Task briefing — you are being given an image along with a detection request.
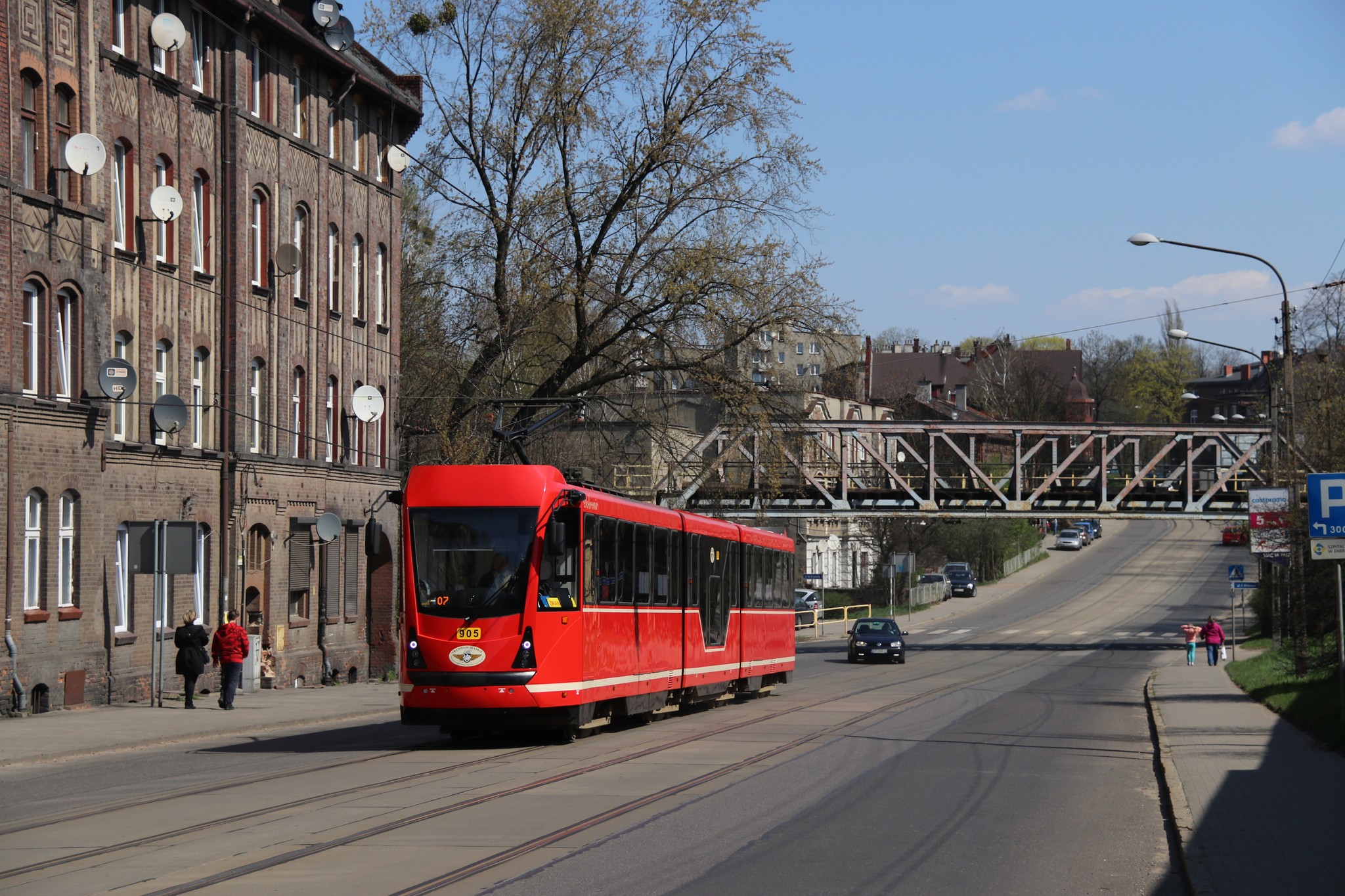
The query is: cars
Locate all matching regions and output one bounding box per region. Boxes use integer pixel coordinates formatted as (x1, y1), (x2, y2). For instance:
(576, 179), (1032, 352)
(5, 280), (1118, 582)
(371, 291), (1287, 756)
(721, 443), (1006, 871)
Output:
(1055, 529), (1082, 550)
(1069, 519), (1102, 546)
(795, 595), (815, 631)
(946, 570), (977, 597)
(917, 572), (952, 601)
(1221, 525), (1249, 546)
(847, 617), (909, 664)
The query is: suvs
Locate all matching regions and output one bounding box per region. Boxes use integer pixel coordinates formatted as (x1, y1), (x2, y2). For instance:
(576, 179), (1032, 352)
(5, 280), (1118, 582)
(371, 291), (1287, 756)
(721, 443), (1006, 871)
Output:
(795, 588), (823, 619)
(942, 561), (974, 576)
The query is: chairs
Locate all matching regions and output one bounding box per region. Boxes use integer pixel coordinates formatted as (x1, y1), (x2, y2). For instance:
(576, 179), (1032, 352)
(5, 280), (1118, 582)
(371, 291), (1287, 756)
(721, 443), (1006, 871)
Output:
(860, 624), (869, 631)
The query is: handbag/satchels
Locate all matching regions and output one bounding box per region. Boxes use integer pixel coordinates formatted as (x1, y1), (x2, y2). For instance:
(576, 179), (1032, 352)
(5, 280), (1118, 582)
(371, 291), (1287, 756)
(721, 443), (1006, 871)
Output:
(201, 647), (210, 665)
(1220, 642), (1227, 660)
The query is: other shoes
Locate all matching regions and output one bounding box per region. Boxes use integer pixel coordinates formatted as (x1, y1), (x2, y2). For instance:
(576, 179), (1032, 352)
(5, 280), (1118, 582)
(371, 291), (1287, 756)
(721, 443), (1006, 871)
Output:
(1209, 663), (1213, 666)
(1214, 663), (1217, 665)
(1188, 662), (1191, 665)
(218, 697), (226, 708)
(1191, 662), (1194, 666)
(185, 704), (196, 708)
(225, 704), (235, 710)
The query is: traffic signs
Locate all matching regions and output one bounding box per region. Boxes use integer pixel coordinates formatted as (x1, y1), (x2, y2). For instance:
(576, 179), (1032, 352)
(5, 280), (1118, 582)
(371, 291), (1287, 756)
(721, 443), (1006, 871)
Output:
(1307, 473), (1345, 538)
(1228, 564), (1244, 580)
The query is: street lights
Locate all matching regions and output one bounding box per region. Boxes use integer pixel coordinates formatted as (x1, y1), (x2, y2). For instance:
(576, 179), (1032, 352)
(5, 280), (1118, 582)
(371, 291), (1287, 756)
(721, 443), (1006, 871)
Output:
(1128, 232), (1310, 682)
(1169, 329), (1280, 651)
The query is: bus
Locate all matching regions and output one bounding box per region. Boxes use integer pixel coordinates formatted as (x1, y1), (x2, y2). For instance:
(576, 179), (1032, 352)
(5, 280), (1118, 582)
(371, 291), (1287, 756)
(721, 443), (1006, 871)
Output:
(365, 396), (796, 746)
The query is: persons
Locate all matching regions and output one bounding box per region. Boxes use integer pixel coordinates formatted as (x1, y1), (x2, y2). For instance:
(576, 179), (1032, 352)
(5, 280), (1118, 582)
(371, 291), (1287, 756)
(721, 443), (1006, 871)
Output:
(476, 552), (511, 588)
(211, 609), (249, 710)
(1181, 624), (1202, 666)
(1200, 615), (1225, 666)
(174, 609), (210, 709)
(876, 623), (894, 635)
